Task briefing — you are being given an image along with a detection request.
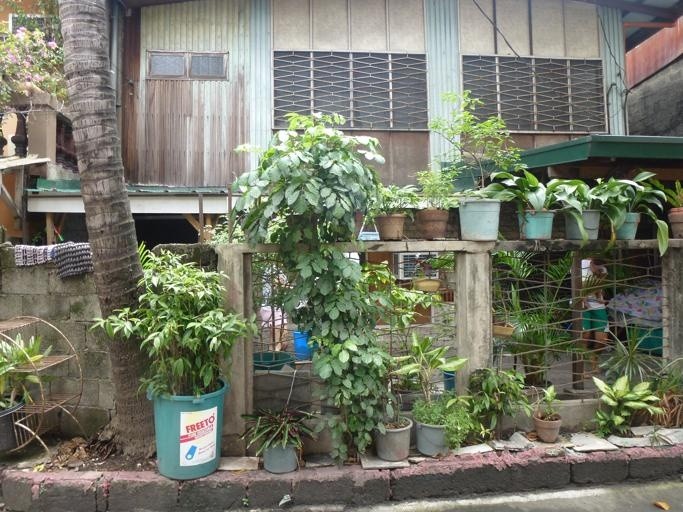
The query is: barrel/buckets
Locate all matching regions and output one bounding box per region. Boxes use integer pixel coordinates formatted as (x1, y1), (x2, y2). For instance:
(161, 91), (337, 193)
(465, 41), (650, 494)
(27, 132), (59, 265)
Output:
(443, 370), (455, 392)
(373, 416), (413, 462)
(564, 209), (601, 240)
(0, 394), (27, 459)
(413, 416), (450, 458)
(458, 198), (501, 242)
(152, 377), (228, 481)
(263, 442), (299, 474)
(293, 331), (321, 362)
(252, 350), (296, 373)
(515, 209), (558, 240)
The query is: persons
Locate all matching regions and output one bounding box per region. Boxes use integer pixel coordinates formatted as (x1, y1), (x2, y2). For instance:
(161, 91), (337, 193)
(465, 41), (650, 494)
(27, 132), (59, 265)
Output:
(573, 258), (607, 376)
(579, 259), (610, 310)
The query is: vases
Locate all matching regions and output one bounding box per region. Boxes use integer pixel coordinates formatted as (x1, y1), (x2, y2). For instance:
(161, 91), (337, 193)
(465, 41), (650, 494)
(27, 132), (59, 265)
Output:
(442, 369), (455, 391)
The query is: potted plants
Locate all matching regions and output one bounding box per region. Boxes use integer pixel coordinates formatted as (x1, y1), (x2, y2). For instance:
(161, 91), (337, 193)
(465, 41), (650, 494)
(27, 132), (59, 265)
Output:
(586, 171), (670, 258)
(490, 161), (590, 240)
(84, 237), (261, 483)
(427, 89), (526, 242)
(409, 163), (464, 239)
(1, 329), (66, 455)
(531, 384), (562, 444)
(412, 390), (476, 458)
(664, 179), (681, 239)
(551, 175), (603, 240)
(238, 402), (325, 477)
(368, 182), (419, 240)
(348, 163), (379, 240)
(365, 347), (422, 461)
